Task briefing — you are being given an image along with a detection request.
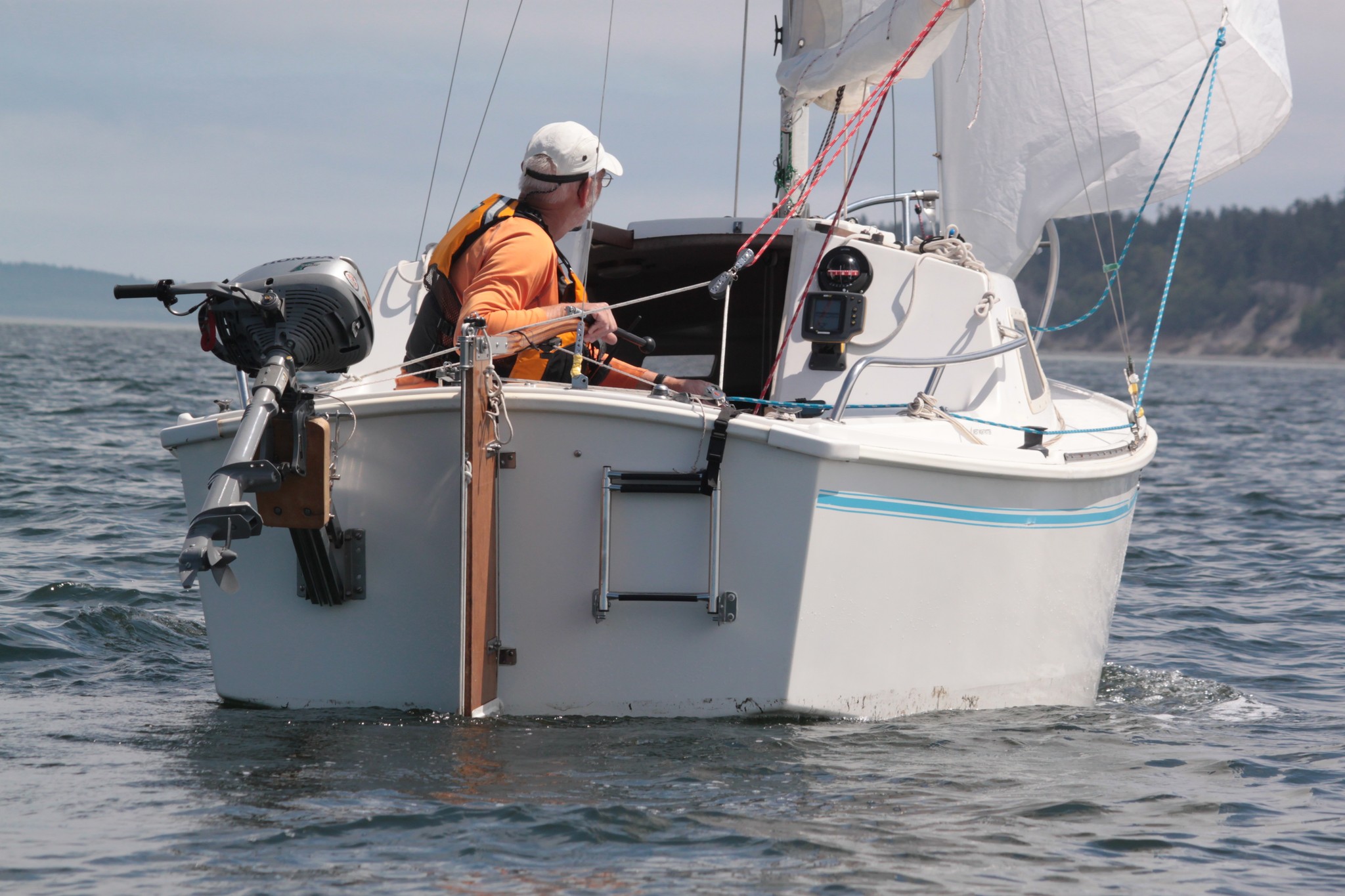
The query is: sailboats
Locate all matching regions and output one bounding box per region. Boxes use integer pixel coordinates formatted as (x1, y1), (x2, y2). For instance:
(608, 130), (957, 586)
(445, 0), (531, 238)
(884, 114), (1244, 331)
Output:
(114, 0), (1294, 722)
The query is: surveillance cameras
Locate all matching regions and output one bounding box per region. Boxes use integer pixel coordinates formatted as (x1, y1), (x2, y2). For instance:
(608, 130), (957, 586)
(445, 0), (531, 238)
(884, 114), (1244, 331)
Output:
(818, 246), (873, 295)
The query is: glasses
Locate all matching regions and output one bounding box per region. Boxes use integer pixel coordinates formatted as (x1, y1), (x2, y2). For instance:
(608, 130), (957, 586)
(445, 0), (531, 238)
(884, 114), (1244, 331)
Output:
(577, 171), (613, 199)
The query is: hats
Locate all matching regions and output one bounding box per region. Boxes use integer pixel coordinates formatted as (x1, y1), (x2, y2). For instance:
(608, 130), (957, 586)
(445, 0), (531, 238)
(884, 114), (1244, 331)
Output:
(520, 121), (624, 184)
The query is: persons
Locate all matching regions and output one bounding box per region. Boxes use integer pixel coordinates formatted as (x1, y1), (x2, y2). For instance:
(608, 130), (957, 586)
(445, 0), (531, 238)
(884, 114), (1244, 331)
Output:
(394, 121), (734, 413)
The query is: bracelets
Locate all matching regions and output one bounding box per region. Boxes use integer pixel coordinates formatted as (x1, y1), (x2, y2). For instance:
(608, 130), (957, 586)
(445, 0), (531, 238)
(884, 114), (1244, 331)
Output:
(653, 373), (668, 388)
